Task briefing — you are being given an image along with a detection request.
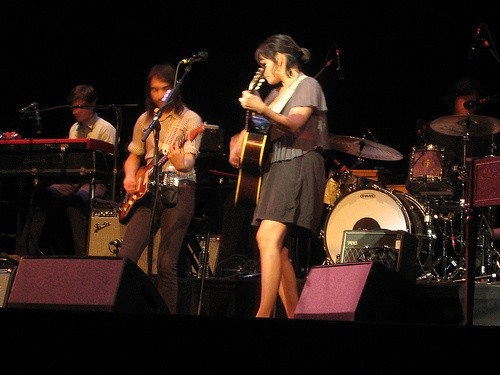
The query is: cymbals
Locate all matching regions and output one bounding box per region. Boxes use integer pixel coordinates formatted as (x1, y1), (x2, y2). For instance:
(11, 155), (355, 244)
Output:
(327, 134), (405, 162)
(430, 113), (500, 136)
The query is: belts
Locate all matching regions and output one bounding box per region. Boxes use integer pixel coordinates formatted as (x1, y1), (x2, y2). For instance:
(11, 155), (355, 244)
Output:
(179, 180), (195, 189)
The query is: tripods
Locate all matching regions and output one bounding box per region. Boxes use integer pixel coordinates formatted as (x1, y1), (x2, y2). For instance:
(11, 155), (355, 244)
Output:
(416, 186), (466, 282)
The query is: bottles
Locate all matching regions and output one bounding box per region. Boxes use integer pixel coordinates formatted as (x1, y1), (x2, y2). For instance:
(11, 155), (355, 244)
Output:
(163, 166), (179, 186)
(197, 246), (209, 278)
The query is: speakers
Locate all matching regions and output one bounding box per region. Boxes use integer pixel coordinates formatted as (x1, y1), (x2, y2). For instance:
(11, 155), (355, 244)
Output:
(191, 185), (252, 271)
(87, 208), (161, 276)
(0, 269), (12, 307)
(339, 230), (419, 283)
(7, 255), (170, 315)
(293, 261), (413, 323)
(185, 234), (235, 277)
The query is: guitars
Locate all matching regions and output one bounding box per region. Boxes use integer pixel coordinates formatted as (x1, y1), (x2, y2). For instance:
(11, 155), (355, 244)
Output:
(233, 62), (274, 208)
(118, 121), (208, 224)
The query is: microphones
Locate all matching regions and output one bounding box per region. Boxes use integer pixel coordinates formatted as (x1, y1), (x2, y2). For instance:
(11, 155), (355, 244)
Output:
(416, 122), (425, 143)
(464, 96), (491, 110)
(180, 52), (208, 64)
(336, 48), (344, 79)
(19, 102), (38, 113)
(472, 26), (481, 50)
(109, 239), (123, 247)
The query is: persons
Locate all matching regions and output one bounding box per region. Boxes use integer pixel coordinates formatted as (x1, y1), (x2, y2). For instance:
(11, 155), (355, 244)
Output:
(427, 82), (486, 160)
(118, 65), (203, 315)
(230, 34), (327, 318)
(8, 84), (120, 256)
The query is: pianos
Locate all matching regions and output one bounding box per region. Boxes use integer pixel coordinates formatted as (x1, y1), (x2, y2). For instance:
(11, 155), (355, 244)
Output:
(0, 137), (116, 257)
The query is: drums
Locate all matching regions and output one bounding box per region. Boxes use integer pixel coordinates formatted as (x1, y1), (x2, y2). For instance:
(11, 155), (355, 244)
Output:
(407, 143), (463, 198)
(322, 168), (373, 207)
(322, 185), (434, 278)
